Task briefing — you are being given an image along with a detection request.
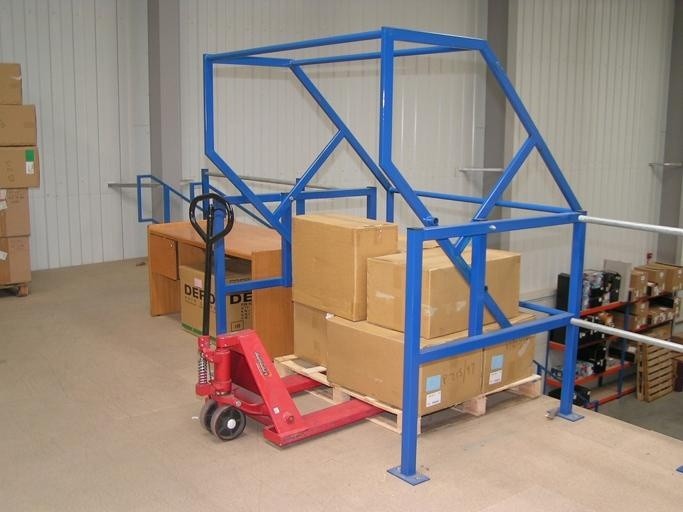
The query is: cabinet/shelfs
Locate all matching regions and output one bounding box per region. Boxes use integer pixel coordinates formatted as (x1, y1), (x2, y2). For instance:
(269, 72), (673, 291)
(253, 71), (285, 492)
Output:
(546, 292), (677, 410)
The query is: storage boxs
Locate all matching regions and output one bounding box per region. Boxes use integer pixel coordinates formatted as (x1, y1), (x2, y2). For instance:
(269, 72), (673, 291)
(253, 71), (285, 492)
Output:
(178, 259), (252, 344)
(292, 213), (536, 416)
(0, 63), (40, 291)
(607, 262), (683, 350)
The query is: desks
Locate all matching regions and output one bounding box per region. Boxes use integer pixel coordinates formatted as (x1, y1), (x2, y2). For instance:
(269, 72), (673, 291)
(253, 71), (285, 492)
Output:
(147, 220), (294, 362)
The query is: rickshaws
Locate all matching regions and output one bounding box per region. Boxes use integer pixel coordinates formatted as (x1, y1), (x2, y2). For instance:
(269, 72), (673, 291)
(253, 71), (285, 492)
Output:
(188, 193), (387, 448)
